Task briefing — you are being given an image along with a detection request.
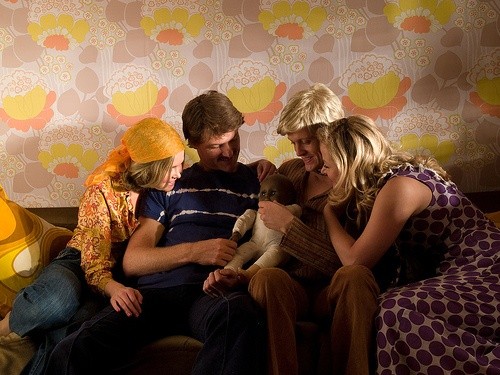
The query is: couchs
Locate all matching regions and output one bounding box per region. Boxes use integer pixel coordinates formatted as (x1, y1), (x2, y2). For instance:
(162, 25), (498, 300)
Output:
(25, 190), (500, 375)
